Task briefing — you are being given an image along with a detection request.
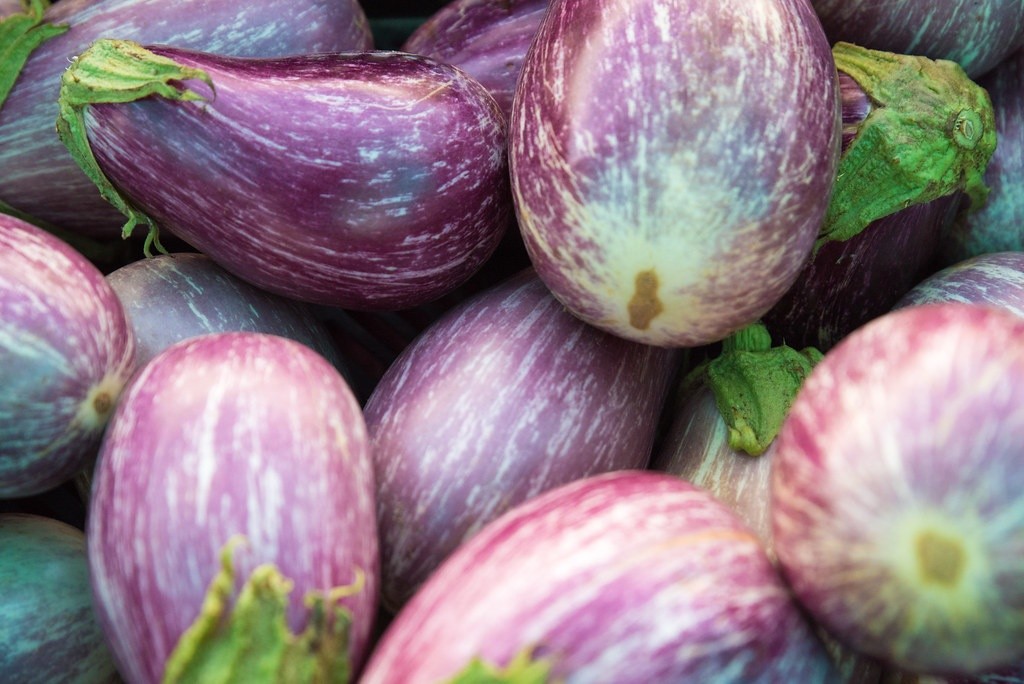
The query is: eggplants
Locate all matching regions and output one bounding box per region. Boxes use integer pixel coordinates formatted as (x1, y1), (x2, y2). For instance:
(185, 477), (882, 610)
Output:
(0, 0), (1024, 684)
